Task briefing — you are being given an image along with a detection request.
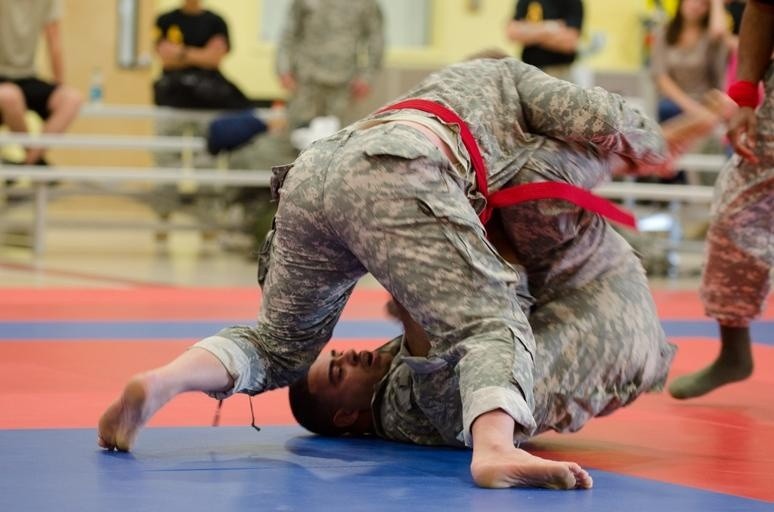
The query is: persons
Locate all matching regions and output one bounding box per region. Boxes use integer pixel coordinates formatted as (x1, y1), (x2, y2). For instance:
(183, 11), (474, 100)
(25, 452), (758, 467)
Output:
(142, 0), (234, 266)
(506, 0), (584, 82)
(708, 0), (766, 162)
(269, 0), (385, 132)
(0, 1), (84, 191)
(96, 44), (673, 493)
(284, 94), (734, 449)
(666, 0), (773, 400)
(641, 0), (731, 125)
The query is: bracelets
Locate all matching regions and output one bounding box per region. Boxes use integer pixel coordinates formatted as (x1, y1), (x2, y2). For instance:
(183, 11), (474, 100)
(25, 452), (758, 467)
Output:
(724, 79), (760, 109)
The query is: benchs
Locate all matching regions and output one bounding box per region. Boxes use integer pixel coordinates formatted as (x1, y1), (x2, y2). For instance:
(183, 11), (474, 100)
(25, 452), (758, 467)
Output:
(593, 154), (725, 245)
(1, 105), (285, 252)
